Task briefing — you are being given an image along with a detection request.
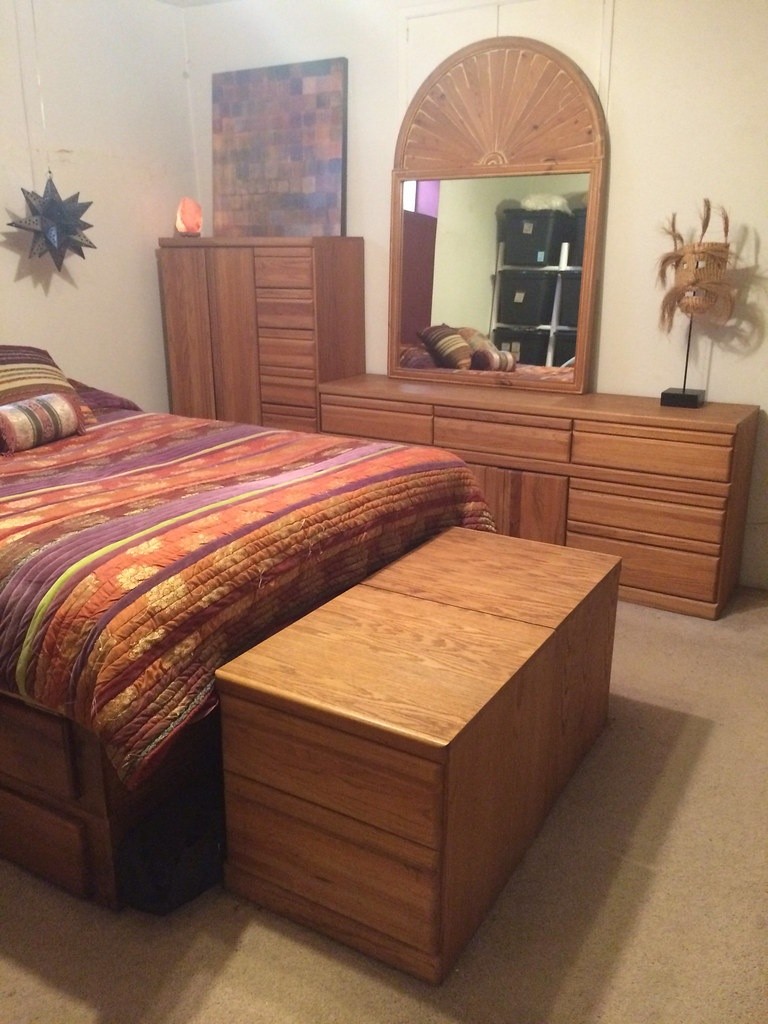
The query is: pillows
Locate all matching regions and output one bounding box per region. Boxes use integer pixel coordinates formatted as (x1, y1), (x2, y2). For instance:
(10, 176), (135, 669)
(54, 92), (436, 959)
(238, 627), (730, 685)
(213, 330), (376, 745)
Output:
(0, 394), (88, 455)
(400, 343), (439, 370)
(0, 344), (97, 427)
(471, 349), (516, 372)
(418, 323), (473, 370)
(454, 327), (498, 353)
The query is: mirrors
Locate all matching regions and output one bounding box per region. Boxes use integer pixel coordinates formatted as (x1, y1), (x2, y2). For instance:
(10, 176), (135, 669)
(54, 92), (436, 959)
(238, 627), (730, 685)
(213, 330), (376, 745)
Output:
(385, 162), (606, 396)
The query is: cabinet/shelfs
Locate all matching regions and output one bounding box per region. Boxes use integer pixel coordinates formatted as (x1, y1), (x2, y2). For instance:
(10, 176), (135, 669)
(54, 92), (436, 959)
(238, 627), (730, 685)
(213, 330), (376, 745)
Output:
(320, 371), (760, 618)
(155, 234), (364, 435)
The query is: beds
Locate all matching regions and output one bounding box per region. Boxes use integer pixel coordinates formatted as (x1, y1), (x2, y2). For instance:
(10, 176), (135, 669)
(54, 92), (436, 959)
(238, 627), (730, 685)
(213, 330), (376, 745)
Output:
(0, 375), (498, 917)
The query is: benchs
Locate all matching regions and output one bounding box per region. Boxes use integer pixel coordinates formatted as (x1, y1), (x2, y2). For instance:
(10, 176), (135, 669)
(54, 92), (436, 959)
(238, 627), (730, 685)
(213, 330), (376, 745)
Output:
(215, 525), (621, 987)
